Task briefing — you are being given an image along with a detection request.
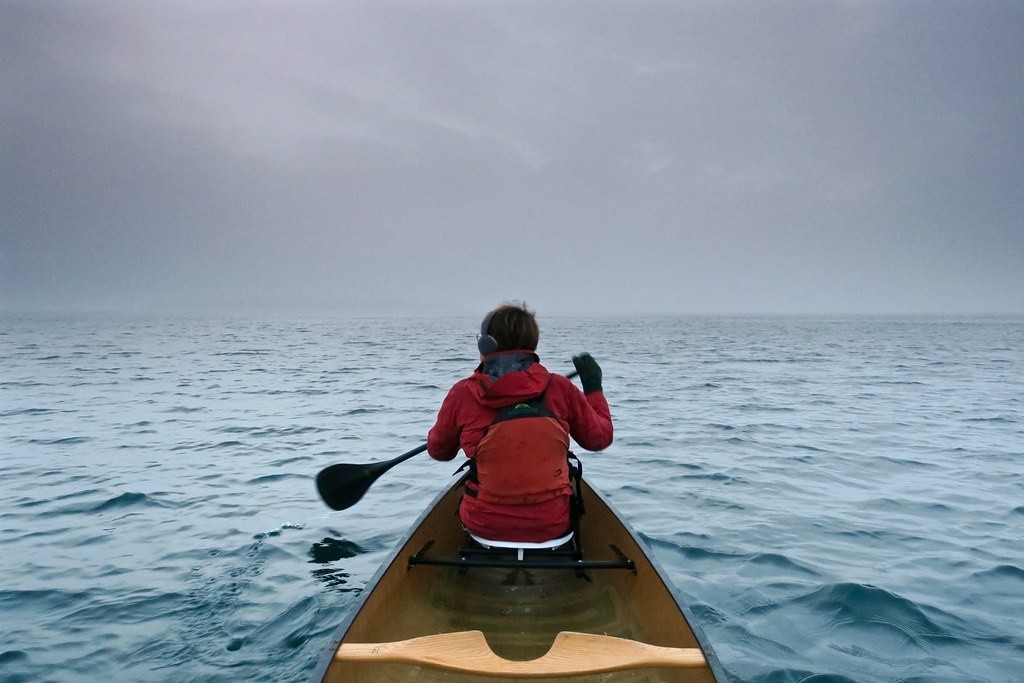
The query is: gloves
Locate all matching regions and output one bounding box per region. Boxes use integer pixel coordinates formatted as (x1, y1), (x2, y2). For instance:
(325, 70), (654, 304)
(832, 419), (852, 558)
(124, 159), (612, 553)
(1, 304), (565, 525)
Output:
(573, 351), (602, 393)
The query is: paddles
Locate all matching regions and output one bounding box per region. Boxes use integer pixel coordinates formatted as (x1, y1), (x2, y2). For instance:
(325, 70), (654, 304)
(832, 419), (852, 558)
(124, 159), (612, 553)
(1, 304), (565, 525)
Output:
(310, 367), (582, 515)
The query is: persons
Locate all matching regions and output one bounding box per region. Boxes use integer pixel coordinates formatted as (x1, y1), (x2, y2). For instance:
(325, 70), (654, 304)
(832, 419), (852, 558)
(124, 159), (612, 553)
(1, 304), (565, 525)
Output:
(427, 305), (613, 554)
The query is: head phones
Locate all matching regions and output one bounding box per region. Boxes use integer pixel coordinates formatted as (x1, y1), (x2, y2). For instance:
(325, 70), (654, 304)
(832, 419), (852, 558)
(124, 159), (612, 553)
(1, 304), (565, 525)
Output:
(478, 309), (497, 356)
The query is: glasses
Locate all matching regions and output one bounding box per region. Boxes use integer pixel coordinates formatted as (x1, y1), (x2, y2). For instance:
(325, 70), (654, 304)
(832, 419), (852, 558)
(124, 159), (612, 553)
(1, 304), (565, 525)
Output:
(476, 333), (483, 342)
